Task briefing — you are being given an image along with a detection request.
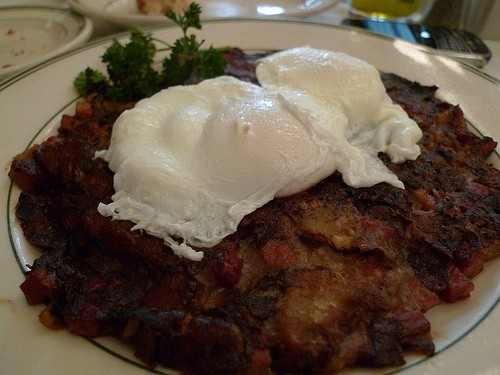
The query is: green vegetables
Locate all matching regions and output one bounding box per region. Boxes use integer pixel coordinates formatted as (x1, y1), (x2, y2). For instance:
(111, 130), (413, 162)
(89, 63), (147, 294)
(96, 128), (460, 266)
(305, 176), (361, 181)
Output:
(75, 2), (237, 98)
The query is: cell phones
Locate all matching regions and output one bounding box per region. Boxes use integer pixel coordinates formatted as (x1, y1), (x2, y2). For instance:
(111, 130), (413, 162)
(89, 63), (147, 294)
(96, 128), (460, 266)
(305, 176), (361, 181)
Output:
(342, 18), (492, 68)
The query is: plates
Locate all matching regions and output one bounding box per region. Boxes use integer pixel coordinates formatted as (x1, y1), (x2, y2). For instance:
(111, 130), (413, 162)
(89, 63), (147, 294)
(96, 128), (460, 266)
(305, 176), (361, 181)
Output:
(1, 0), (92, 78)
(72, 0), (351, 29)
(0, 18), (499, 374)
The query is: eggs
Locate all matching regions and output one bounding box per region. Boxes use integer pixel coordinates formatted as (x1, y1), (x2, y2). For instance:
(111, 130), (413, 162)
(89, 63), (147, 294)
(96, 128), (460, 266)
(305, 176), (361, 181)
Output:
(255, 49), (421, 163)
(94, 76), (404, 261)
